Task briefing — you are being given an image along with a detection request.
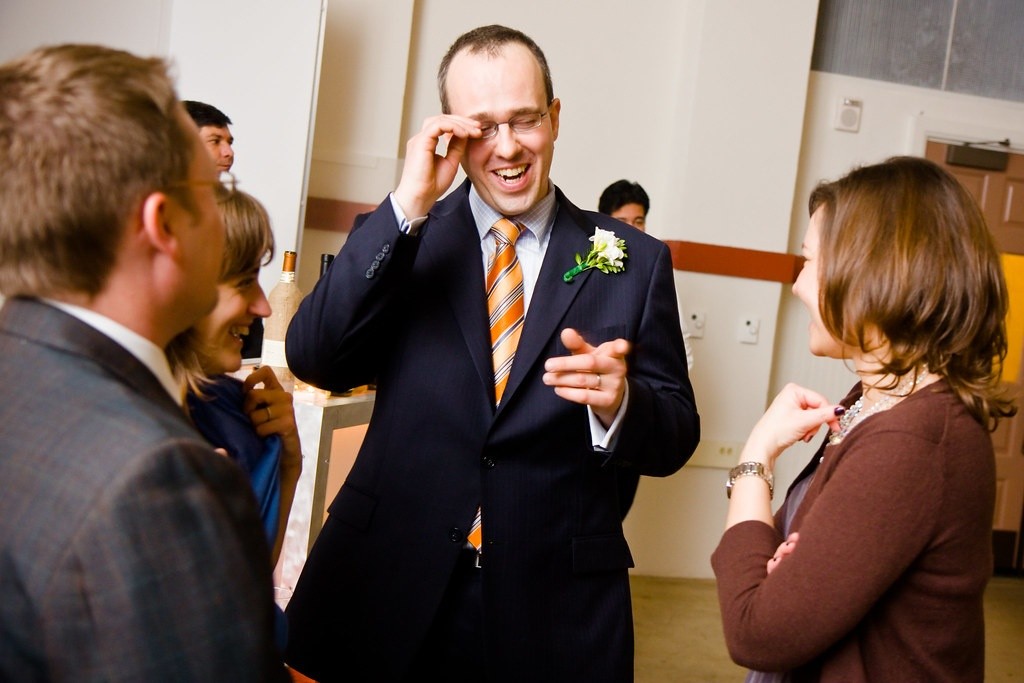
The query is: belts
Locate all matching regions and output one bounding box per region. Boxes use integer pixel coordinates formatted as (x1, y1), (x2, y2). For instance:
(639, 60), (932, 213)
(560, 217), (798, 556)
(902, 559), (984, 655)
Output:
(452, 547), (492, 571)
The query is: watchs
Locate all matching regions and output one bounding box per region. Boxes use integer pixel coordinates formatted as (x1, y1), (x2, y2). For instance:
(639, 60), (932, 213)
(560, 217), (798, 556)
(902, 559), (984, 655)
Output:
(726, 462), (774, 502)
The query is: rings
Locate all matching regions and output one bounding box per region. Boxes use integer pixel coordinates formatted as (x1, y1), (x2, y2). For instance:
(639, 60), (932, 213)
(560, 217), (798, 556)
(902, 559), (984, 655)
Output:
(594, 373), (601, 391)
(266, 407), (272, 422)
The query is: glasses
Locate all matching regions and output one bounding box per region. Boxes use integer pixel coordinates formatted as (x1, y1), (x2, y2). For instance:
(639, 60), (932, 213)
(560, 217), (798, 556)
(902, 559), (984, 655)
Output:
(136, 167), (239, 233)
(479, 101), (554, 138)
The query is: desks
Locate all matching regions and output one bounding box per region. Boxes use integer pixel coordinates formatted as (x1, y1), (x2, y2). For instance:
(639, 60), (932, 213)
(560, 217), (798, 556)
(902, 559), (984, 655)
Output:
(234, 368), (375, 613)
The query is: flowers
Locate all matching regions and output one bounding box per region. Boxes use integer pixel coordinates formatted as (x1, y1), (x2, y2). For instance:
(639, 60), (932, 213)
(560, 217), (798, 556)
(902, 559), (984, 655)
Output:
(563, 225), (629, 282)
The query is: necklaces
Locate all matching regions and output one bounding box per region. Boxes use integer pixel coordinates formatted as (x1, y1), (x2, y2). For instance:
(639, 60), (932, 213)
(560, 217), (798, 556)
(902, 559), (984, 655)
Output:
(818, 363), (930, 445)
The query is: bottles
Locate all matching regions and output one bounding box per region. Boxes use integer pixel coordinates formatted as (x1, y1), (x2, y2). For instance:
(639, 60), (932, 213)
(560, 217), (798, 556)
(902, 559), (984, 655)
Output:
(260, 250), (306, 394)
(320, 253), (334, 278)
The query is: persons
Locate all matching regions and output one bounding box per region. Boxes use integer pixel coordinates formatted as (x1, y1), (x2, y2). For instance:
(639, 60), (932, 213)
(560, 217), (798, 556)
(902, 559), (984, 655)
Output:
(0, 45), (287, 683)
(597, 179), (650, 234)
(180, 100), (264, 383)
(710, 156), (1017, 683)
(283, 25), (701, 683)
(163, 184), (303, 683)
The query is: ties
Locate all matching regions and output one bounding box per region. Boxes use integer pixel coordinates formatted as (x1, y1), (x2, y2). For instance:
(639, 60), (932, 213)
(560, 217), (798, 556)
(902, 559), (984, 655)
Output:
(466, 218), (527, 555)
